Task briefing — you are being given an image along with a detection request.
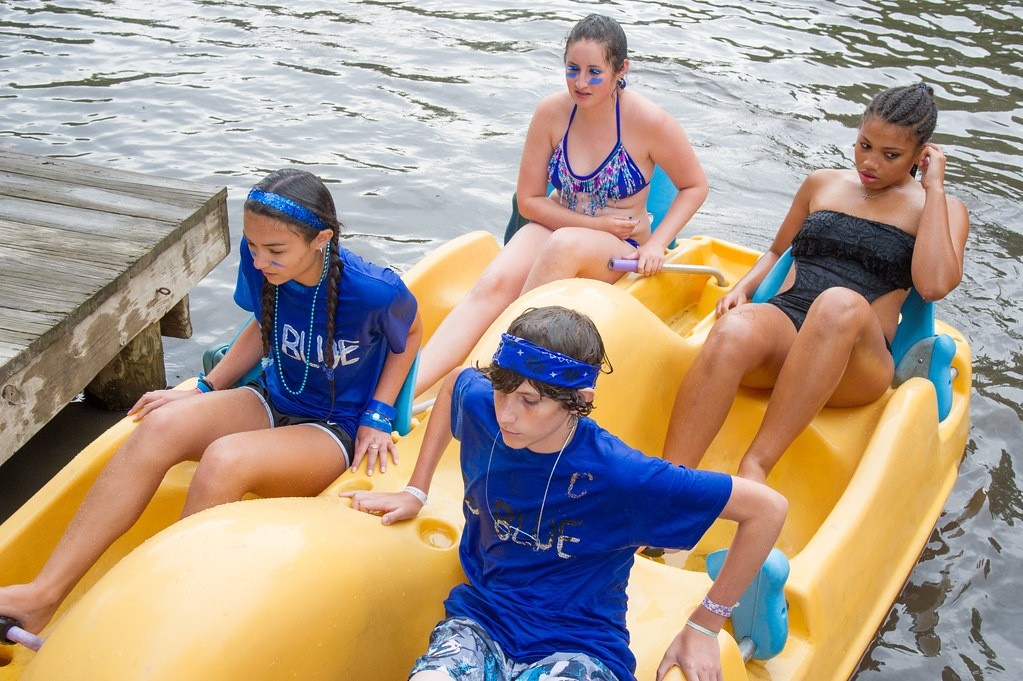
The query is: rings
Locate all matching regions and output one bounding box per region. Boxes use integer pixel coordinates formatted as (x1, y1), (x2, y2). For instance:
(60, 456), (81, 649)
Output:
(369, 445), (379, 449)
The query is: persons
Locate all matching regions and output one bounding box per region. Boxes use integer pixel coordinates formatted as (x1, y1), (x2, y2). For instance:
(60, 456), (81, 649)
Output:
(636, 79), (968, 555)
(412, 13), (709, 402)
(340, 306), (788, 681)
(0, 169), (423, 636)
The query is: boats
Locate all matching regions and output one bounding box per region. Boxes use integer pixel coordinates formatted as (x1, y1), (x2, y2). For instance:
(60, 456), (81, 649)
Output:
(1, 230), (972, 680)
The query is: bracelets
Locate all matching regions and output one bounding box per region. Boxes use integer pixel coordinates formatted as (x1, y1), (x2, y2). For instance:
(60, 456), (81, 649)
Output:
(685, 620), (719, 638)
(196, 378), (215, 394)
(359, 400), (398, 433)
(404, 486), (427, 504)
(702, 597), (733, 617)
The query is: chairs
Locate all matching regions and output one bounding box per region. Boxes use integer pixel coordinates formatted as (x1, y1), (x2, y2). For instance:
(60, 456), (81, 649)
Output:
(535, 161), (681, 257)
(225, 310), (417, 434)
(754, 246), (956, 425)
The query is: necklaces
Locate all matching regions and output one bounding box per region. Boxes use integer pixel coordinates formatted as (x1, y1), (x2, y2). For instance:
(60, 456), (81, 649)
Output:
(486, 418), (577, 550)
(275, 241), (330, 395)
(861, 184), (901, 202)
